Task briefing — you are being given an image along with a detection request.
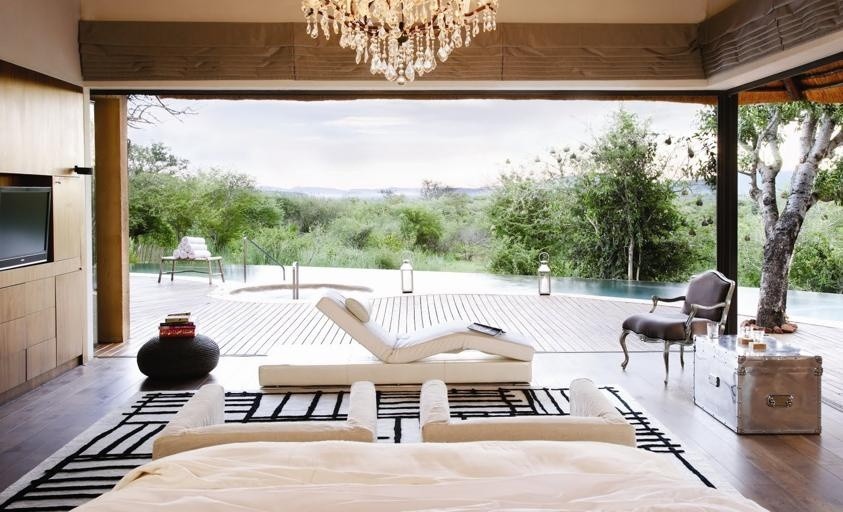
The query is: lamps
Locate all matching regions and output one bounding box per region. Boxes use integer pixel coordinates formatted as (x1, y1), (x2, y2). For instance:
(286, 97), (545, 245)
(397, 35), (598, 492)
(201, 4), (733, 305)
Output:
(298, 0), (500, 90)
(71, 165), (95, 176)
(535, 251), (552, 296)
(399, 260), (414, 294)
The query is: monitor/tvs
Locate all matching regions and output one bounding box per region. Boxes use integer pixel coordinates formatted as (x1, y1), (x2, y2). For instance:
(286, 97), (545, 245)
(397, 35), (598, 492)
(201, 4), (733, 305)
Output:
(0, 186), (52, 271)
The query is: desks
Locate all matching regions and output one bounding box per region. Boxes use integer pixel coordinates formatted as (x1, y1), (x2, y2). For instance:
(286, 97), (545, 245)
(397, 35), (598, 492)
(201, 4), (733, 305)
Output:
(155, 256), (224, 288)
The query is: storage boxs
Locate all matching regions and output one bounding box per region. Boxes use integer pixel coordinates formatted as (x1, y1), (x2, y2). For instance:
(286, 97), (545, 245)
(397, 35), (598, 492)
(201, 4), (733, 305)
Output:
(684, 331), (827, 438)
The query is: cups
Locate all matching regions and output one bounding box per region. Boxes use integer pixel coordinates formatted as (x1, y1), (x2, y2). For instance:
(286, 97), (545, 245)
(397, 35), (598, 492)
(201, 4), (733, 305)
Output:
(706, 322), (765, 346)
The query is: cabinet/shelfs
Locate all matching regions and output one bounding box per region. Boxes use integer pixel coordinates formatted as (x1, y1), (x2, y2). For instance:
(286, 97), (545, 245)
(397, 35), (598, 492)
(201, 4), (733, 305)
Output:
(0, 258), (85, 396)
(22, 176), (82, 262)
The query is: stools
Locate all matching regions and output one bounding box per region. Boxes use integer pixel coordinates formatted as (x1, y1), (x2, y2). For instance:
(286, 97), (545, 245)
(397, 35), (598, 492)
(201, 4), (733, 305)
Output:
(134, 334), (219, 381)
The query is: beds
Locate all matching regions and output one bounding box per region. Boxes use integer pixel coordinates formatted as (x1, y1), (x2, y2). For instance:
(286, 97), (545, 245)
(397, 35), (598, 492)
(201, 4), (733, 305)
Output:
(65, 434), (767, 512)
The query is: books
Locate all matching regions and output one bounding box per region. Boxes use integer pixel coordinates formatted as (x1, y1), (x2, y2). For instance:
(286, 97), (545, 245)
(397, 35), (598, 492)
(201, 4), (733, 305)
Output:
(466, 322), (508, 338)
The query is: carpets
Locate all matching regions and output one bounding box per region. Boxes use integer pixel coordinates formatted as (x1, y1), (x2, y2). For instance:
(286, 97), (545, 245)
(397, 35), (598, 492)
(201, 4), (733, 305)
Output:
(0, 383), (744, 512)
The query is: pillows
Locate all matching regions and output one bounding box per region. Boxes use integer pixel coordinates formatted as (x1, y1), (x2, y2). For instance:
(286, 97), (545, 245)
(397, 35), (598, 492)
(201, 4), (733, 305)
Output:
(343, 292), (371, 324)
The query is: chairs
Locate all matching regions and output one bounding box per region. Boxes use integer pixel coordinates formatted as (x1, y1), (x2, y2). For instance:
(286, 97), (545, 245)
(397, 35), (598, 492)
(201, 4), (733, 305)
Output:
(149, 379), (381, 460)
(614, 267), (735, 391)
(413, 373), (638, 449)
(257, 290), (536, 387)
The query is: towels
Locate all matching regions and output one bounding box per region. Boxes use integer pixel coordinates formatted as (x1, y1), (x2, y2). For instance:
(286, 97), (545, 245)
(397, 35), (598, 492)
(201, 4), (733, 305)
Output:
(171, 235), (211, 261)
